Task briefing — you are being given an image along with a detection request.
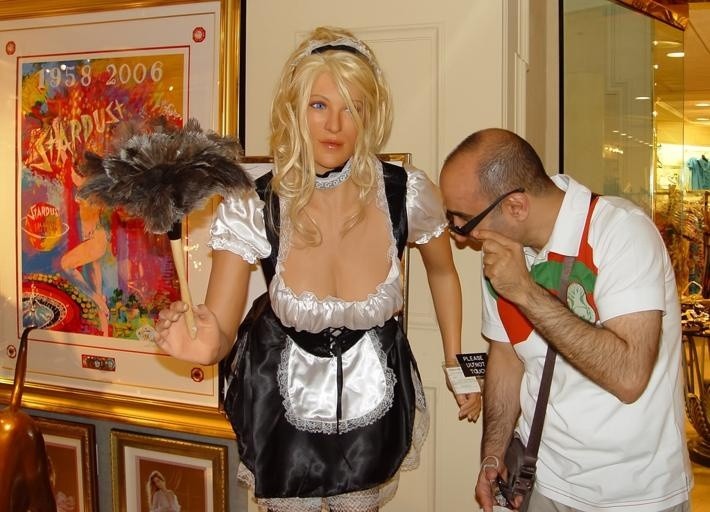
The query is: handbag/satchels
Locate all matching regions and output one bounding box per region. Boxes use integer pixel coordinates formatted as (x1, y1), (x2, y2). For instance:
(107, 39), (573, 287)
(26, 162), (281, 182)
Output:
(490, 436), (548, 512)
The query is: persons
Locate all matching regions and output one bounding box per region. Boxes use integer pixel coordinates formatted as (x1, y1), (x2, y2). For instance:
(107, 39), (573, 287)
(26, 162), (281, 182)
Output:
(439, 128), (695, 512)
(147, 470), (181, 512)
(52, 118), (110, 335)
(153, 26), (481, 512)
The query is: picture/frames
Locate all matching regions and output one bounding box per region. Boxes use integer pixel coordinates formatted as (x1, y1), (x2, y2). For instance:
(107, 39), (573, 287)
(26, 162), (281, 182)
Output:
(106, 430), (231, 512)
(1, 0), (245, 442)
(28, 414), (98, 512)
(241, 154), (414, 338)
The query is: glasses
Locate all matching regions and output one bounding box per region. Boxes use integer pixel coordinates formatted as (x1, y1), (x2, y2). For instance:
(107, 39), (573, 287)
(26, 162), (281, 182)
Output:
(445, 186), (527, 238)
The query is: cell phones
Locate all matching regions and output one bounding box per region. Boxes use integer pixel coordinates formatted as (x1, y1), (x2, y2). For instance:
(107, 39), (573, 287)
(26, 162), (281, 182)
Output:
(492, 473), (516, 509)
